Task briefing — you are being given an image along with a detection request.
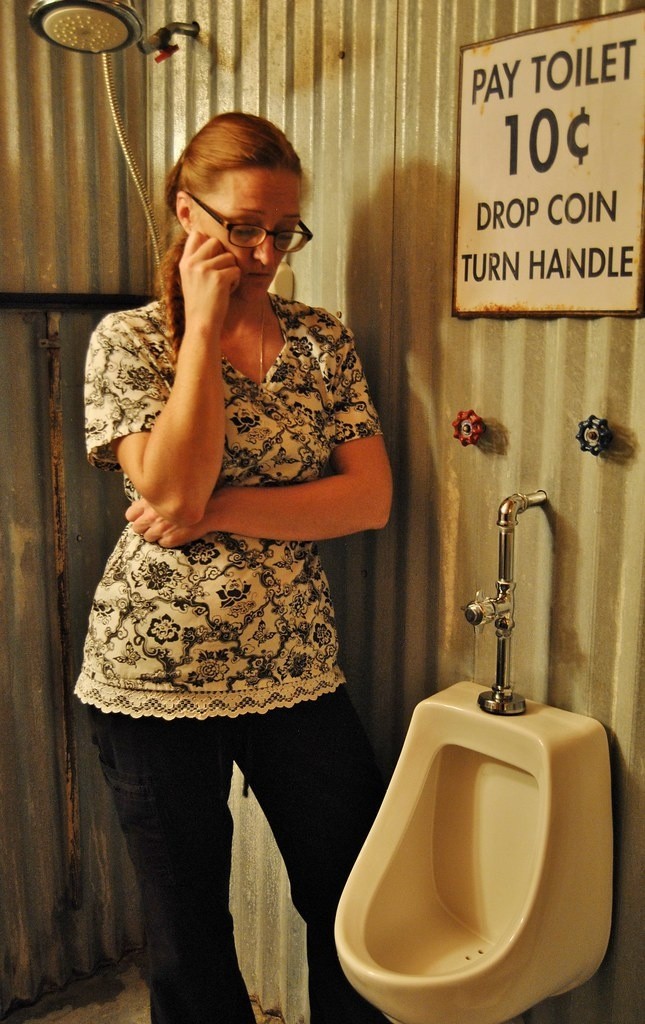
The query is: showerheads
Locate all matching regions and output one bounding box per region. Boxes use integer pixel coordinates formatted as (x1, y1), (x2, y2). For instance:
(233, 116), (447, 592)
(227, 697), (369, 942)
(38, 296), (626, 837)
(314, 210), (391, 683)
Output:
(29, 0), (145, 55)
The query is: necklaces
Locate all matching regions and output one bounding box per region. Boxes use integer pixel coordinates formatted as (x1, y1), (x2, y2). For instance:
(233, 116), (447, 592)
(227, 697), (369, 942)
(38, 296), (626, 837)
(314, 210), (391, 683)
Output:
(220, 293), (265, 414)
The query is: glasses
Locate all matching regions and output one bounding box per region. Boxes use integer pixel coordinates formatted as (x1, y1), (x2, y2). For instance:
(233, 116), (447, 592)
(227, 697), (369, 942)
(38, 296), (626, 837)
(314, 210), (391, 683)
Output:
(186, 191), (313, 254)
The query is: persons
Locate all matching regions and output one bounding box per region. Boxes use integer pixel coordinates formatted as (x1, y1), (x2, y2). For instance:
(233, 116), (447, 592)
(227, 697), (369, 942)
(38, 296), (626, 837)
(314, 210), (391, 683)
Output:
(74, 113), (392, 1024)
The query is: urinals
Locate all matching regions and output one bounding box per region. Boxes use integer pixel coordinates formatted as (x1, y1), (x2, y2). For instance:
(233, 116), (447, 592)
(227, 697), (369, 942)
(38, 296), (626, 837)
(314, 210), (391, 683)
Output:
(335, 682), (614, 1017)
